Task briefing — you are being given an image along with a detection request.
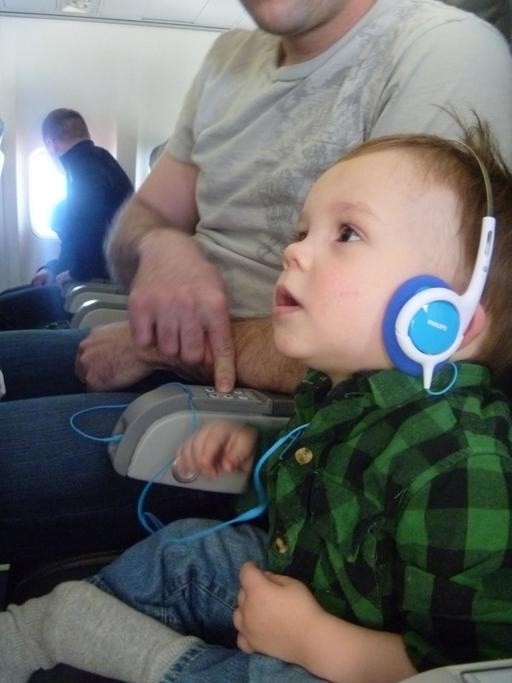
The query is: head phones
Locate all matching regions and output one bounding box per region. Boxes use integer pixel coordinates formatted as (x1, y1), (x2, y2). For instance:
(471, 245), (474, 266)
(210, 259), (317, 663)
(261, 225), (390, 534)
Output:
(382, 140), (497, 390)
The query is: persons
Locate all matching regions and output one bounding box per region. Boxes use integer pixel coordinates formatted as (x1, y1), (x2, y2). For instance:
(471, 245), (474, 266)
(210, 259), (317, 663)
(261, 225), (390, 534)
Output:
(0, 97), (512, 683)
(1, 106), (135, 326)
(0, 2), (512, 552)
